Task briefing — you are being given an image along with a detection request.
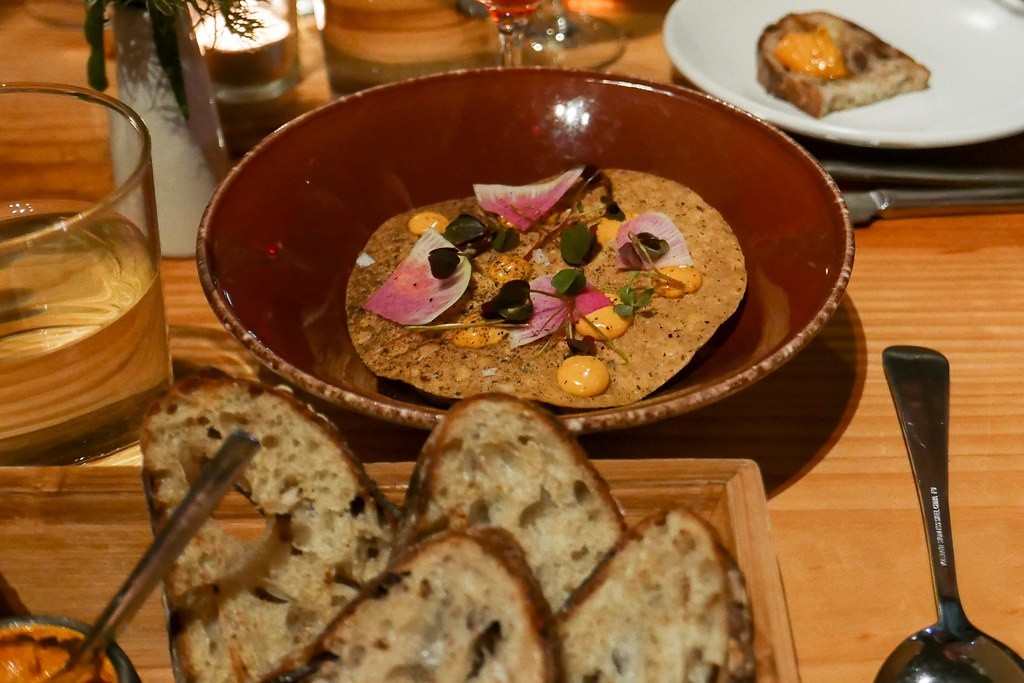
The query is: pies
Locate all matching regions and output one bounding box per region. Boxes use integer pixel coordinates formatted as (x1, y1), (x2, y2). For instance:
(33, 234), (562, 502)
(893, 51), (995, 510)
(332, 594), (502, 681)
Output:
(343, 167), (747, 411)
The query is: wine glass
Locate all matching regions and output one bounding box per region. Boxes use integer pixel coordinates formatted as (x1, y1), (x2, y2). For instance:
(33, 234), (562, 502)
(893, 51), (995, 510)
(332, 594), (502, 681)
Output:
(502, 0), (626, 77)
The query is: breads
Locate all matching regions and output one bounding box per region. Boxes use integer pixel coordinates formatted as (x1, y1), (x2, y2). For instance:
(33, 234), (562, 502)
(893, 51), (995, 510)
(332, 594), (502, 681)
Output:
(139, 376), (756, 683)
(755, 10), (931, 118)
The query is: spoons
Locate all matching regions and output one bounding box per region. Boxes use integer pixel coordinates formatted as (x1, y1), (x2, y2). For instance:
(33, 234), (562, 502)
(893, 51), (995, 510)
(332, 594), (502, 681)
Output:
(873, 344), (1024, 683)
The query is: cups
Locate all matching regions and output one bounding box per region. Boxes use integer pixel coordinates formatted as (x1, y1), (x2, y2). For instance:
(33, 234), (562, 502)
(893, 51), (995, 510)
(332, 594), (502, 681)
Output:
(1, 81), (176, 466)
(173, 0), (304, 98)
(321, 0), (506, 102)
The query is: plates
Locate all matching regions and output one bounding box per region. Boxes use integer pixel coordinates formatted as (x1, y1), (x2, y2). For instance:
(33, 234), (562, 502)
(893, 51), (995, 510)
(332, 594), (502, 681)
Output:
(661, 0), (1024, 149)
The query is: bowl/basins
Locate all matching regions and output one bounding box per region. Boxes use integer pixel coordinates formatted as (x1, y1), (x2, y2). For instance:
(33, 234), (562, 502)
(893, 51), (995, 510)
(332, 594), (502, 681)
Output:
(195, 66), (855, 432)
